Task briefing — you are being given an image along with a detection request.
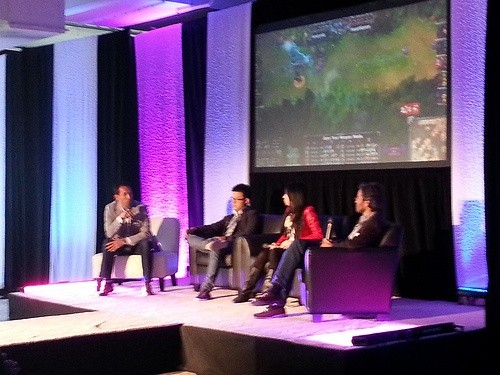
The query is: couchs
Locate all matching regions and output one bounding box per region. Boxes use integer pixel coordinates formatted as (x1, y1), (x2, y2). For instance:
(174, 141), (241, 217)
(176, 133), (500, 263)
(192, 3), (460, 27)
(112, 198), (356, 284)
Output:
(186, 212), (405, 322)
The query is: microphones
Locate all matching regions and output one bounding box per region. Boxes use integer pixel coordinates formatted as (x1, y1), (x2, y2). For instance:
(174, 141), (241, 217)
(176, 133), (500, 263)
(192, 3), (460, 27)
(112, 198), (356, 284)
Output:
(325, 219), (332, 239)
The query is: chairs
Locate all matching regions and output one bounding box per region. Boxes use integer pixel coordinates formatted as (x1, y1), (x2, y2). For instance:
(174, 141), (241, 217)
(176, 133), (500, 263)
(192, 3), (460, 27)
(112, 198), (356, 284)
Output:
(92, 217), (180, 292)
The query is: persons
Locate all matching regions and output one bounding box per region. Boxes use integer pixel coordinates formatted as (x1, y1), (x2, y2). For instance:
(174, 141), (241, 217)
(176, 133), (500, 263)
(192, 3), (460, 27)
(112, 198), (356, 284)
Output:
(233, 185), (323, 302)
(186, 183), (260, 301)
(250, 183), (386, 319)
(97, 185), (157, 296)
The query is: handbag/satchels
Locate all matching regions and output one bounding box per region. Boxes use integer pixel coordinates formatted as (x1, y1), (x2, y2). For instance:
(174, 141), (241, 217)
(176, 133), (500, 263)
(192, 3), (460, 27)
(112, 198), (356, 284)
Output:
(115, 221), (163, 256)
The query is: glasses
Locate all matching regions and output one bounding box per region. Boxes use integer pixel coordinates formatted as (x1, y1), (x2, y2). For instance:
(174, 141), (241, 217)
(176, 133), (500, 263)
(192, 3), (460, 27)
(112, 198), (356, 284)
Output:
(230, 197), (244, 201)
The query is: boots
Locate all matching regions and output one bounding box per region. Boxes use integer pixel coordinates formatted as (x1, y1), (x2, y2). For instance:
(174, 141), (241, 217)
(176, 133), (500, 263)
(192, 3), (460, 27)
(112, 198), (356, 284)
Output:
(250, 268), (276, 301)
(233, 267), (259, 303)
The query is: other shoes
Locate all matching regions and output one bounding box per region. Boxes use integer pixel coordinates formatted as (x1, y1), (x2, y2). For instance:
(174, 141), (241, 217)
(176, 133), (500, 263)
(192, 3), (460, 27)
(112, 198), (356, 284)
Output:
(197, 290), (211, 301)
(208, 240), (228, 251)
(252, 290), (285, 307)
(98, 280), (113, 296)
(142, 285), (152, 296)
(253, 307), (285, 320)
